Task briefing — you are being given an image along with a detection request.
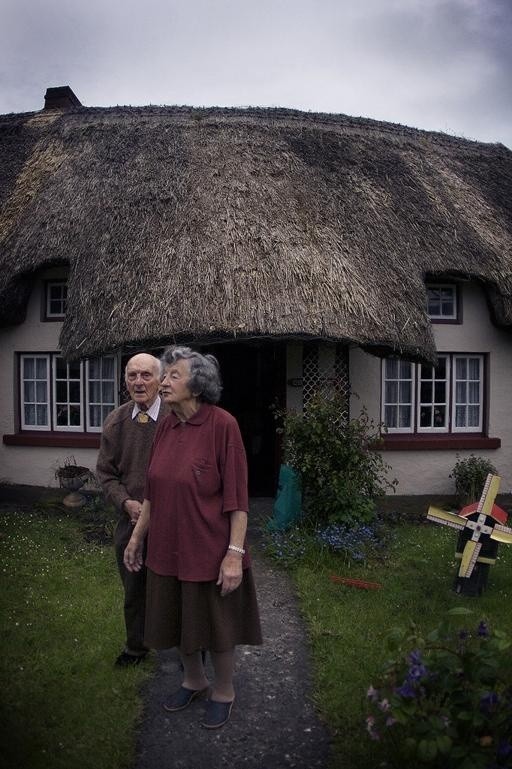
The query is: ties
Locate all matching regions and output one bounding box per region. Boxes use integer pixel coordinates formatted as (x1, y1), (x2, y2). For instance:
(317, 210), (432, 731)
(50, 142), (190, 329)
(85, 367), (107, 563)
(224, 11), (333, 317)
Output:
(136, 412), (150, 424)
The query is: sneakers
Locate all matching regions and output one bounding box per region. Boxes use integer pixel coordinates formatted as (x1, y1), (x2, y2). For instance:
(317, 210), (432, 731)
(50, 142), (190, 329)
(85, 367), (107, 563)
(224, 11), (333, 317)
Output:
(202, 694), (234, 728)
(163, 685), (200, 712)
(115, 644), (148, 668)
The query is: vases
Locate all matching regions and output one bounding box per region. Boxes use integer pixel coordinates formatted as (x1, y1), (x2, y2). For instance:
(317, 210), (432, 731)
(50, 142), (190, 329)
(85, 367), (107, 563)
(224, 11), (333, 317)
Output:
(56, 465), (92, 508)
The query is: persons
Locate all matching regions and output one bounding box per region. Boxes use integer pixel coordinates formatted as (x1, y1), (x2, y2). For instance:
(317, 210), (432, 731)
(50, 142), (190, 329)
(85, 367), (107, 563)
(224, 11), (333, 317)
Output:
(123, 346), (263, 728)
(95, 354), (208, 675)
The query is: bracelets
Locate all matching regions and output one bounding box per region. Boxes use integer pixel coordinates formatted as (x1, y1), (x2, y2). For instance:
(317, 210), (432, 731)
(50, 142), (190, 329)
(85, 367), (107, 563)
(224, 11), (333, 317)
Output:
(228, 544), (246, 555)
